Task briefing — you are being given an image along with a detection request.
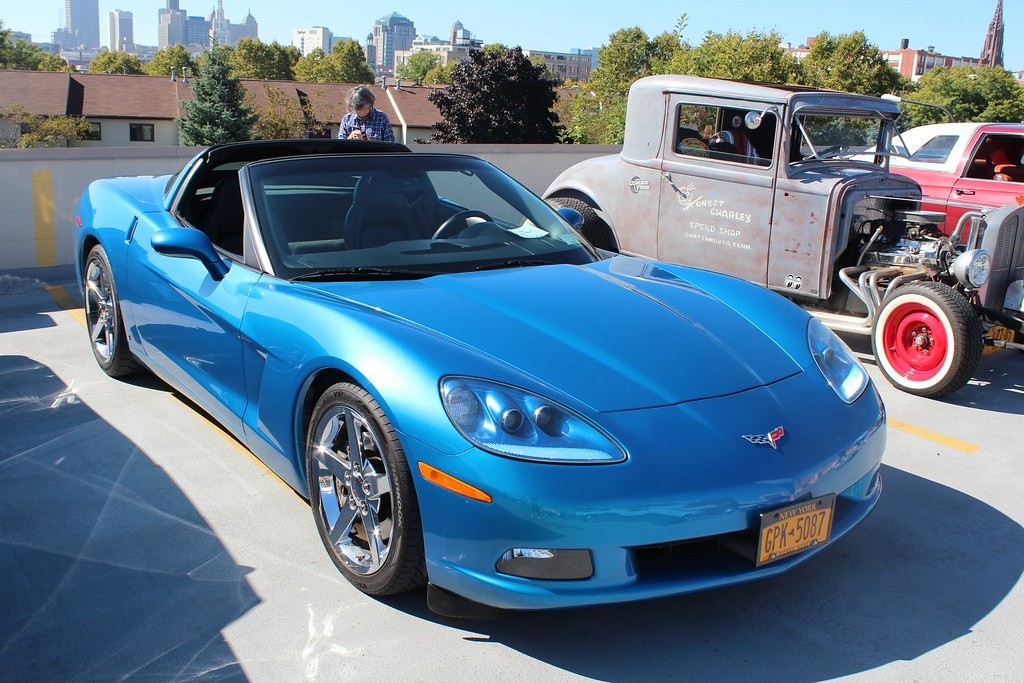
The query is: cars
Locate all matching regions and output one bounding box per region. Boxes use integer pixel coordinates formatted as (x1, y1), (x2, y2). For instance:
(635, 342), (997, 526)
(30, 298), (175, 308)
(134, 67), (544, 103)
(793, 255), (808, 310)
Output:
(519, 73), (1024, 400)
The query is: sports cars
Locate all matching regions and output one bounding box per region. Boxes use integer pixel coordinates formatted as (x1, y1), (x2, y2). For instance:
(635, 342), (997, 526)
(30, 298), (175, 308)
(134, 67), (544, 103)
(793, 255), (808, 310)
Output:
(72, 137), (888, 622)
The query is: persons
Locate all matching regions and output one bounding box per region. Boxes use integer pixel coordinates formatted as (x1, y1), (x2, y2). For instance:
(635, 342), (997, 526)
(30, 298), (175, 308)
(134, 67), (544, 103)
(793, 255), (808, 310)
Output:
(338, 86), (396, 143)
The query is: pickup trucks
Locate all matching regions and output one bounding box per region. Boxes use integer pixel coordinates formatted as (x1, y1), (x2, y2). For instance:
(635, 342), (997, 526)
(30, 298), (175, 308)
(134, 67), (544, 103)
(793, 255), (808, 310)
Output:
(851, 122), (1024, 259)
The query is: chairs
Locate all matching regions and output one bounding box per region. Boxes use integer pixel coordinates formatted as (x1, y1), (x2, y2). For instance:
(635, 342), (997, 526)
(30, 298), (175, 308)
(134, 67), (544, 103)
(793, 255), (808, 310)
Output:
(209, 178), (293, 252)
(994, 164), (1024, 182)
(346, 173), (422, 250)
(680, 127), (760, 165)
